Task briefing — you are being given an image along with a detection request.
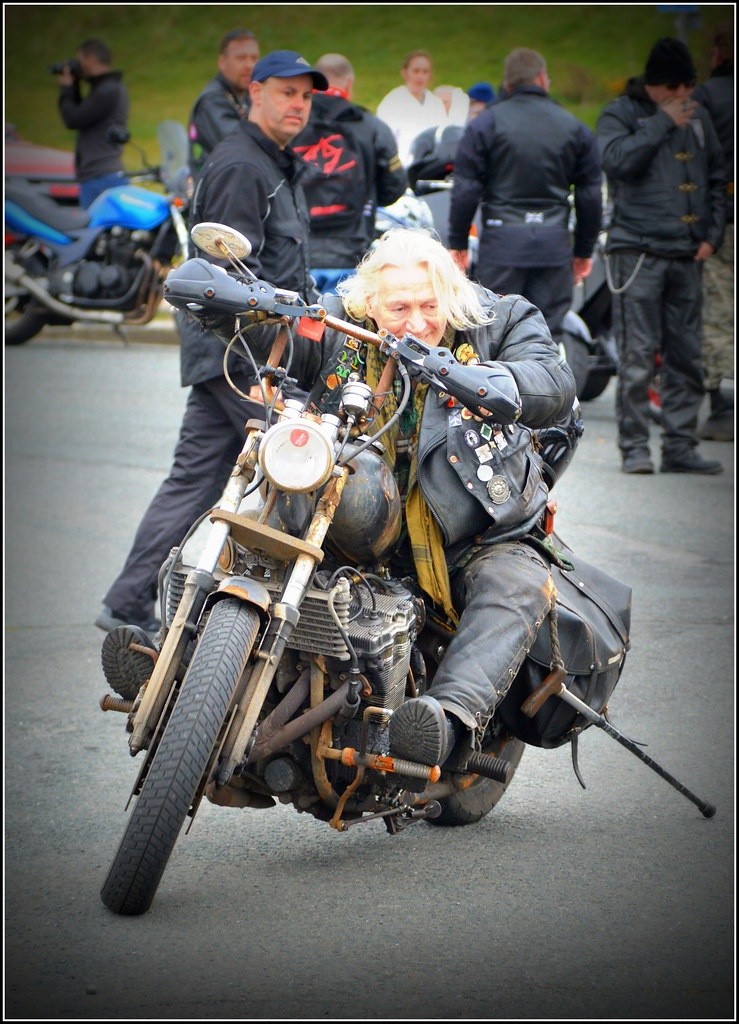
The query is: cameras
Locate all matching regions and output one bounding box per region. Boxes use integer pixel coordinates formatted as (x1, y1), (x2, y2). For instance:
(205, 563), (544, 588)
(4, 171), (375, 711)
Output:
(51, 59), (83, 79)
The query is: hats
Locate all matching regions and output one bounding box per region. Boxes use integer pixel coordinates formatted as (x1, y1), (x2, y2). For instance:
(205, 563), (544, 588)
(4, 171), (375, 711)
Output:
(468, 82), (494, 101)
(642, 37), (699, 85)
(251, 51), (328, 90)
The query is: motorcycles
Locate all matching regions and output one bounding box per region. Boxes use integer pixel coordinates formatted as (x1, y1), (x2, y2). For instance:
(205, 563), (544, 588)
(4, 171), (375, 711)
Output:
(3, 116), (193, 350)
(411, 170), (619, 403)
(98, 220), (587, 916)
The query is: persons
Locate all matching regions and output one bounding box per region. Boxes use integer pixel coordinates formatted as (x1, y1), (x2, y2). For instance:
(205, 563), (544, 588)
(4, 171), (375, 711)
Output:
(88, 24), (311, 645)
(376, 53), (490, 174)
(280, 52), (407, 300)
(448, 43), (605, 330)
(596, 33), (738, 477)
(47, 40), (137, 208)
(101, 228), (582, 795)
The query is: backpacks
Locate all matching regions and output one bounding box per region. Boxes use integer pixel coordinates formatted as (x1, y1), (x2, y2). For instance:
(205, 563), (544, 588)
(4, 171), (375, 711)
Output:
(494, 546), (630, 787)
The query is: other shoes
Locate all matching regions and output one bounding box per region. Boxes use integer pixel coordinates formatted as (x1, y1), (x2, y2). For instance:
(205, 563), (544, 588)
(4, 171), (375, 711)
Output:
(662, 453), (723, 475)
(390, 696), (455, 794)
(102, 624), (159, 702)
(699, 412), (733, 441)
(95, 606), (162, 639)
(621, 447), (656, 474)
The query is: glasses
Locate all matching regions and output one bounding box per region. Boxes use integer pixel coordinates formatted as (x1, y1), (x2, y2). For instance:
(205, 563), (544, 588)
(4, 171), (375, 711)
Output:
(661, 79), (696, 91)
